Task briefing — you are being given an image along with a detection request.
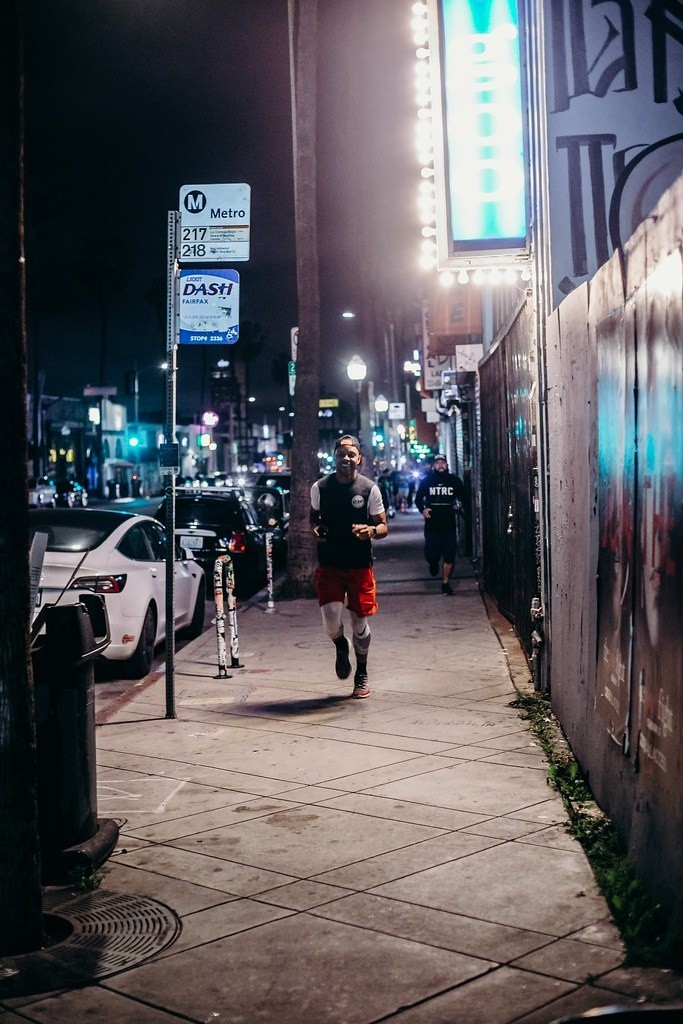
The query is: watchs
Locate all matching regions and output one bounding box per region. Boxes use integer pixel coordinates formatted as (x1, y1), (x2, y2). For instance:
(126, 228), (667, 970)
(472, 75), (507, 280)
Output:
(372, 526), (377, 538)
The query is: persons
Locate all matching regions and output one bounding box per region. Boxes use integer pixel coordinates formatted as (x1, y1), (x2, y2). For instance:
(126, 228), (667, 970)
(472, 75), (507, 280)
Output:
(378, 462), (434, 515)
(414, 454), (467, 595)
(309, 435), (388, 699)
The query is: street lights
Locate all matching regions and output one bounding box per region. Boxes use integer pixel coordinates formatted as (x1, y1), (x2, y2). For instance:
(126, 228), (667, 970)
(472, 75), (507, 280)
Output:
(347, 353), (368, 475)
(374, 394), (389, 470)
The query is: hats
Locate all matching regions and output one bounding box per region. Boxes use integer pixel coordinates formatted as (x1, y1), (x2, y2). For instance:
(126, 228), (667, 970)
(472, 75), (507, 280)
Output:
(434, 454), (447, 463)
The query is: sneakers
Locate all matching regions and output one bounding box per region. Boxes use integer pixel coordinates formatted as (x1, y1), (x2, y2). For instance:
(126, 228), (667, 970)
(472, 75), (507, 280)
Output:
(335, 636), (352, 680)
(335, 435), (362, 466)
(353, 670), (371, 698)
(442, 583), (455, 595)
(430, 563), (440, 576)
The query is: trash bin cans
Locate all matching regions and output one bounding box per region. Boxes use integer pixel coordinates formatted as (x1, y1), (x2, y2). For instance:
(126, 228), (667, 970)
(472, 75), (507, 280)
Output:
(29, 592), (121, 891)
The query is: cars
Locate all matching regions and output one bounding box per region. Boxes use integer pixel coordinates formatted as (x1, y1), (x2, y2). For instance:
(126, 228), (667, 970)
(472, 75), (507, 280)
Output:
(27, 508), (208, 676)
(26, 476), (88, 507)
(154, 471), (291, 585)
